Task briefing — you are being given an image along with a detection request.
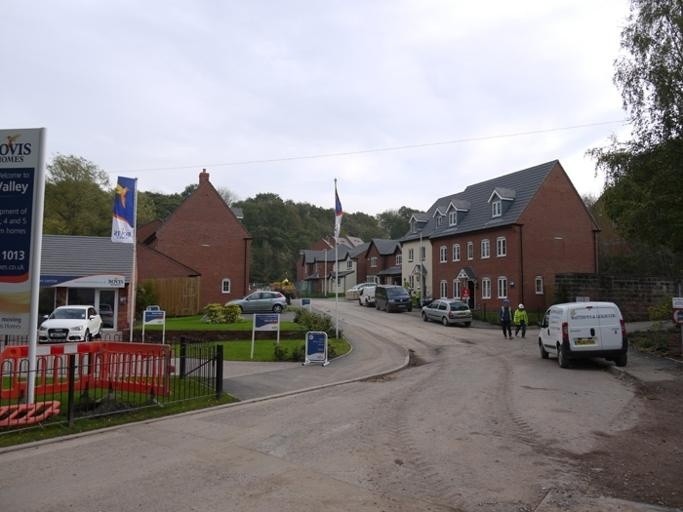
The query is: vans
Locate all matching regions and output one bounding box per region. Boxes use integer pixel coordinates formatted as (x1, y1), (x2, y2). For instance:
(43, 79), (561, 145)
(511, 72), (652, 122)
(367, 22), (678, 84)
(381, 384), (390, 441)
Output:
(536, 300), (633, 371)
(374, 285), (413, 314)
(357, 286), (375, 306)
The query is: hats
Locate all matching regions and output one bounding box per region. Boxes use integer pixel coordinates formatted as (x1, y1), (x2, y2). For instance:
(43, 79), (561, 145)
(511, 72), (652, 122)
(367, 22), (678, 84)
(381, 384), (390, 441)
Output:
(518, 303), (524, 309)
(503, 300), (510, 304)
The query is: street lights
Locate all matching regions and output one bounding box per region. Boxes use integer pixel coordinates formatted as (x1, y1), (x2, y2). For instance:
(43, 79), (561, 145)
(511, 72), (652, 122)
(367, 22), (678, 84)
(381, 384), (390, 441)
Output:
(414, 227), (425, 308)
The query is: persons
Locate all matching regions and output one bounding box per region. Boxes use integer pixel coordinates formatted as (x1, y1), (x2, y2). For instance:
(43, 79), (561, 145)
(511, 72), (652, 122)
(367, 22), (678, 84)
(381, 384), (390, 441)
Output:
(500, 300), (514, 340)
(461, 286), (470, 305)
(513, 303), (527, 337)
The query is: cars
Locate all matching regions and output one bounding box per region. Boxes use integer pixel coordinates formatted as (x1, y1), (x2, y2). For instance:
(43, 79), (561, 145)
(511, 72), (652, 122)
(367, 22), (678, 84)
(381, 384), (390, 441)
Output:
(417, 295), (472, 328)
(99, 303), (112, 316)
(343, 282), (379, 300)
(37, 303), (104, 344)
(223, 290), (288, 315)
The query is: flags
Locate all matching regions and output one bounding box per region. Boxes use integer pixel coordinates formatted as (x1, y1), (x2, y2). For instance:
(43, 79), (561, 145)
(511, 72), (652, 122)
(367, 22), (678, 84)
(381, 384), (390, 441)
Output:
(335, 186), (342, 242)
(111, 175), (135, 243)
